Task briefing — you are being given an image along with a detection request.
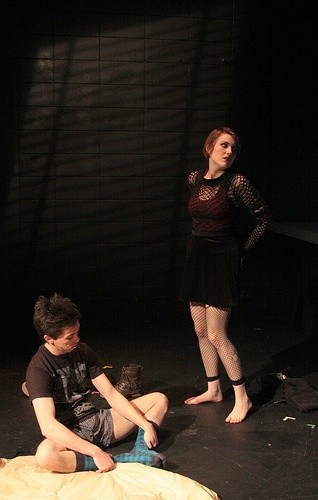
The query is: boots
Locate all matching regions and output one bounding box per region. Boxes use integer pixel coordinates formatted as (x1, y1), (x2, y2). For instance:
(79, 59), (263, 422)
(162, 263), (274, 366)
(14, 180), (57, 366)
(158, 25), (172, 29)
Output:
(100, 363), (142, 399)
(89, 366), (116, 394)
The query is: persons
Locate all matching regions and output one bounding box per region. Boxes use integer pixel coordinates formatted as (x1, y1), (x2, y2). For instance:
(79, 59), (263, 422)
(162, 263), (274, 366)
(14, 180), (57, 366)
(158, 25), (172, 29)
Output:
(25, 294), (170, 473)
(183, 126), (275, 424)
